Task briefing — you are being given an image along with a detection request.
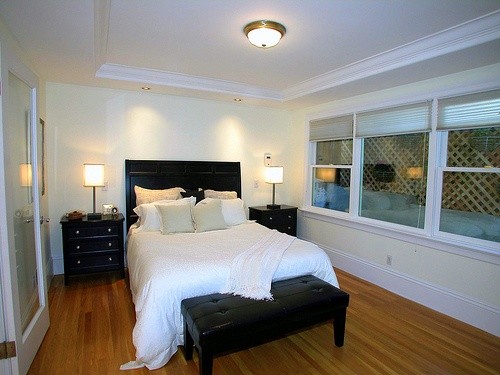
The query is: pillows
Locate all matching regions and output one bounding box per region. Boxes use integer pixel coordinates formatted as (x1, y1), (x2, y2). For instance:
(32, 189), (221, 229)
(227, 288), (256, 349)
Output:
(327, 183), (416, 211)
(133, 185), (257, 235)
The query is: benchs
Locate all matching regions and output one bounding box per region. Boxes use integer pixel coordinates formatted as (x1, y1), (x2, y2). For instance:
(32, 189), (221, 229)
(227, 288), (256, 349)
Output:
(181, 275), (350, 375)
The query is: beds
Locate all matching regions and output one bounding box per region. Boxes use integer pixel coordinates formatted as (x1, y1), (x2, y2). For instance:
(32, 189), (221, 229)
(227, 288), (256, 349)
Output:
(334, 164), (500, 243)
(120, 159), (341, 370)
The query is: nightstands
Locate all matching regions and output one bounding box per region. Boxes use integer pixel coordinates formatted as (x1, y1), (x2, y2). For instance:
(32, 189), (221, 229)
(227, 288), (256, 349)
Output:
(60, 213), (125, 285)
(248, 204), (298, 237)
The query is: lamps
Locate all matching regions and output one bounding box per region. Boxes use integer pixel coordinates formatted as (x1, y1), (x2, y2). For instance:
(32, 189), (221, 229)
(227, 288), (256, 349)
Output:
(83, 164), (105, 220)
(265, 167), (283, 209)
(243, 20), (286, 48)
(316, 168), (336, 208)
(19, 163), (32, 186)
(407, 166), (424, 194)
